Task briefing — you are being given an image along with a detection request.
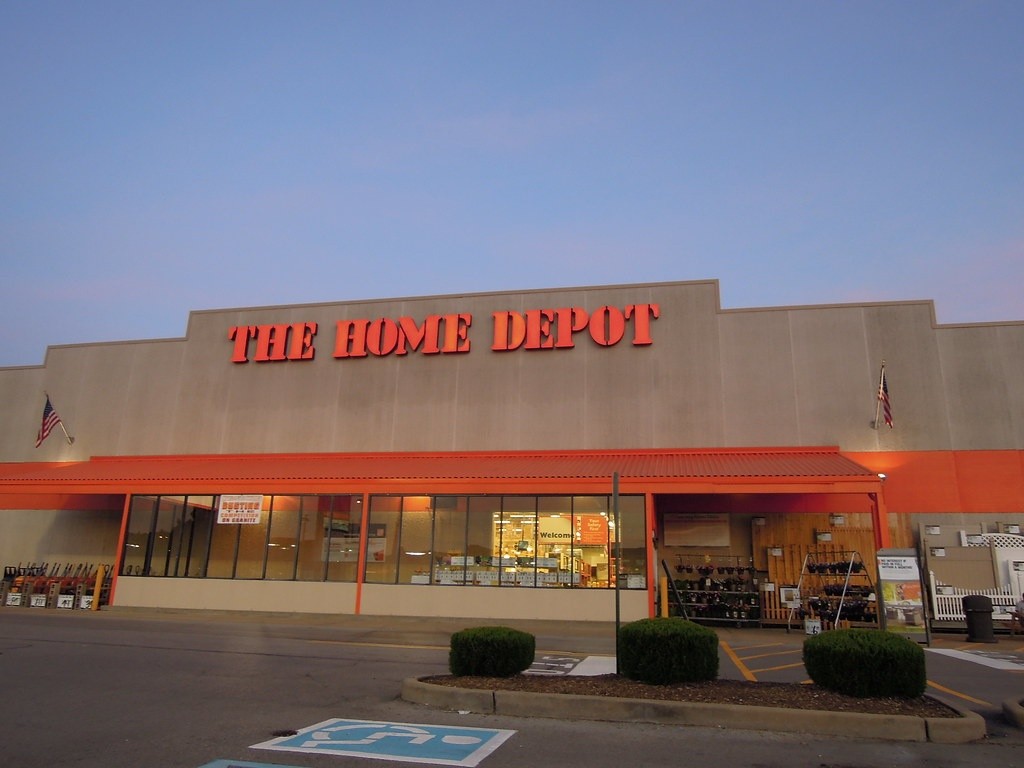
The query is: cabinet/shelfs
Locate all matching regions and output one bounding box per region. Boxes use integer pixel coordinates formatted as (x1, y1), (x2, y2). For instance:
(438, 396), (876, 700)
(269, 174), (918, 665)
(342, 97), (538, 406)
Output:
(668, 588), (762, 629)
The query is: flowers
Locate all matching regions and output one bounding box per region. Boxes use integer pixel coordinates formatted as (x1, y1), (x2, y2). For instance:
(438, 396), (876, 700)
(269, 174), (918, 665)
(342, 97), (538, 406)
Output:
(808, 596), (868, 608)
(796, 606), (837, 618)
(822, 584), (872, 594)
(698, 605), (709, 612)
(701, 592), (722, 599)
(807, 562), (863, 571)
(674, 565), (753, 570)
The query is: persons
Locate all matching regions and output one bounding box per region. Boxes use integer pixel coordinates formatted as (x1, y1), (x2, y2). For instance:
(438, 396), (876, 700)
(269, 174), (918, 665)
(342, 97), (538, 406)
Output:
(1014, 593), (1024, 627)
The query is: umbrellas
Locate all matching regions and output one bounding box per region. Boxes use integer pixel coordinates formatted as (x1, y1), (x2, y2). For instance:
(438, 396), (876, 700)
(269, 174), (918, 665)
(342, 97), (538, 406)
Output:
(18, 562), (113, 578)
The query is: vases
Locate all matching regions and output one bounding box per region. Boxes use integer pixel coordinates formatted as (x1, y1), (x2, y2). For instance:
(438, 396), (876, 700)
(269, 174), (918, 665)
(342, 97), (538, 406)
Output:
(677, 568), (683, 573)
(707, 569), (714, 573)
(839, 613), (877, 623)
(738, 569), (744, 575)
(728, 584), (736, 591)
(811, 605), (864, 613)
(698, 569), (705, 574)
(738, 585), (747, 591)
(727, 569), (734, 574)
(824, 590), (870, 598)
(686, 568), (693, 573)
(796, 613), (836, 622)
(808, 568), (861, 573)
(718, 569), (724, 574)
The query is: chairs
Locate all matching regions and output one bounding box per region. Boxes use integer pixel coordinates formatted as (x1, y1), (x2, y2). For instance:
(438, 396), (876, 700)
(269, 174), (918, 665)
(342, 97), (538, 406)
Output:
(1006, 611), (1024, 641)
(0, 561), (140, 605)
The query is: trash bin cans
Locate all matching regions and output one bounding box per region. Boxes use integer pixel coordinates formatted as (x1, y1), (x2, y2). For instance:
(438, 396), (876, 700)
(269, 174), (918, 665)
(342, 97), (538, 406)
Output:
(962, 595), (998, 643)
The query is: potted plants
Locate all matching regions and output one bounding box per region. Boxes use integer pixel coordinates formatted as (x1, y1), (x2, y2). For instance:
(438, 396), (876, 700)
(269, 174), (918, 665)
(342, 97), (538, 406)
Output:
(670, 580), (761, 620)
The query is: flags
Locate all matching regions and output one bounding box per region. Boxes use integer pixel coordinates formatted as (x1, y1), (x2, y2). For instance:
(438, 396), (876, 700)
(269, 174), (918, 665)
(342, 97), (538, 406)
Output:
(877, 371), (894, 429)
(35, 399), (61, 449)
